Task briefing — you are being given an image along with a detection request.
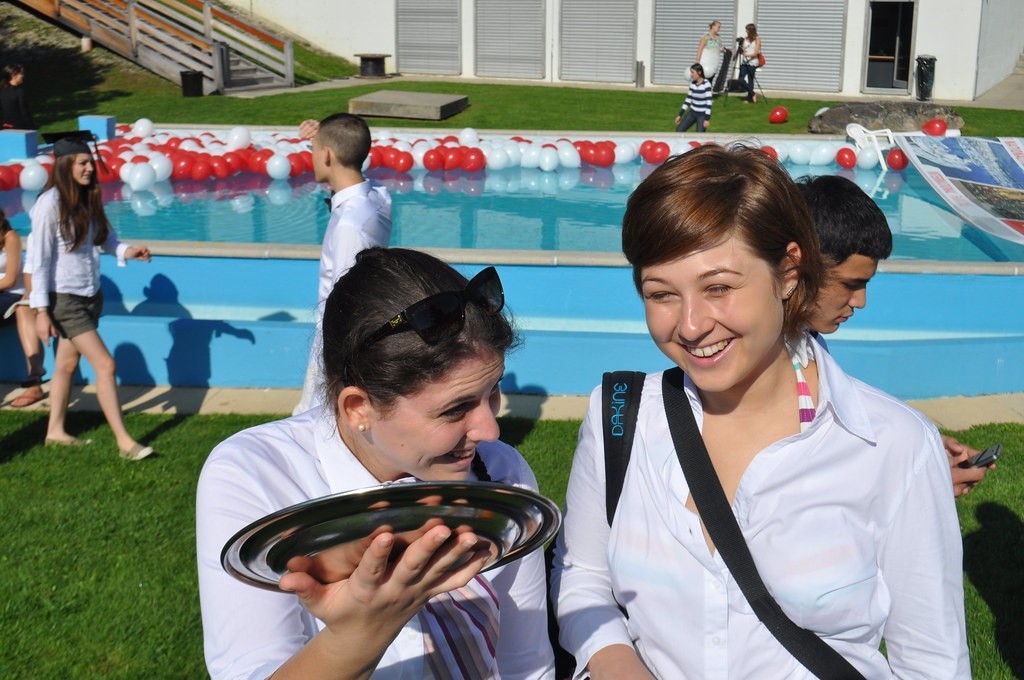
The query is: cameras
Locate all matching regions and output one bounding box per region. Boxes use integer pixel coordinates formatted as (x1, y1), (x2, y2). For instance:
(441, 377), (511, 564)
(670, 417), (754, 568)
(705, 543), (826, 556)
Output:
(737, 38), (744, 44)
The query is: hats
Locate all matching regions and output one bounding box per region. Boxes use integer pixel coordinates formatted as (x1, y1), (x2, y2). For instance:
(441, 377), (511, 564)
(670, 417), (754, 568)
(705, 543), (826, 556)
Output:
(41, 130), (110, 174)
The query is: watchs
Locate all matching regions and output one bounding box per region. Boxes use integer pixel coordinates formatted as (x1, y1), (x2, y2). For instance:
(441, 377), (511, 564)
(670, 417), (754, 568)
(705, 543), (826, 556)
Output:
(32, 306), (47, 314)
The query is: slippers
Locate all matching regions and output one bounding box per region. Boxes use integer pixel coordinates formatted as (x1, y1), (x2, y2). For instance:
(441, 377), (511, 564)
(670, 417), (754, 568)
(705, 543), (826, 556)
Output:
(10, 387), (48, 407)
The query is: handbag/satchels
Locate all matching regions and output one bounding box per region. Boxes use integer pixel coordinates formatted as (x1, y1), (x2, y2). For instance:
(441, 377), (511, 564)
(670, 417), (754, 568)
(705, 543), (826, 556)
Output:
(756, 52), (765, 68)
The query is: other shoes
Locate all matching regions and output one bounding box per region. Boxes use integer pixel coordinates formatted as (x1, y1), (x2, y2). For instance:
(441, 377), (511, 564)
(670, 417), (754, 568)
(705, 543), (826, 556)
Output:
(753, 93), (758, 104)
(45, 437), (93, 446)
(743, 100), (749, 103)
(118, 441), (153, 462)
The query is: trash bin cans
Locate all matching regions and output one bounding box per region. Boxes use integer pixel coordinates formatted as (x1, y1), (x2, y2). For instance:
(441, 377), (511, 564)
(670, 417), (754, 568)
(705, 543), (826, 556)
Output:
(180, 71), (203, 97)
(915, 55), (937, 101)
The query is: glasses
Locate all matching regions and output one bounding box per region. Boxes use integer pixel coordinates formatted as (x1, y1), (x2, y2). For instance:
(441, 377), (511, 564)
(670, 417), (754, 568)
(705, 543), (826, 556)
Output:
(342, 265), (504, 388)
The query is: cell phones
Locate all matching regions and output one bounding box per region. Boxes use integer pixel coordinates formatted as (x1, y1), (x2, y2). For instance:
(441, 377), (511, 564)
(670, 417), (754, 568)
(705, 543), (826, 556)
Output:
(953, 443), (1002, 470)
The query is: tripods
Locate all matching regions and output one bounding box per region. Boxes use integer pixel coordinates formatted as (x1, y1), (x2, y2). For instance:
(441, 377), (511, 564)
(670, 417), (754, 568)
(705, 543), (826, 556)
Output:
(724, 43), (769, 109)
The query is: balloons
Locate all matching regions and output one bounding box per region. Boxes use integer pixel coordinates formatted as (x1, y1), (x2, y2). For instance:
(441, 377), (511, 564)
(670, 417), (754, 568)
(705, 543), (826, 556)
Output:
(921, 119), (947, 136)
(0, 117), (909, 188)
(769, 105), (788, 123)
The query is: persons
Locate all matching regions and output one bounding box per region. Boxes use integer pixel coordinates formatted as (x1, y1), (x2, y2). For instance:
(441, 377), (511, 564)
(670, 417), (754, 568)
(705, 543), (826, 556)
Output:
(551, 140), (973, 680)
(793, 176), (995, 497)
(195, 246), (556, 680)
(697, 21), (732, 87)
(0, 206), (47, 409)
(737, 24), (761, 104)
(27, 130), (154, 460)
(292, 111), (392, 416)
(675, 63), (713, 133)
